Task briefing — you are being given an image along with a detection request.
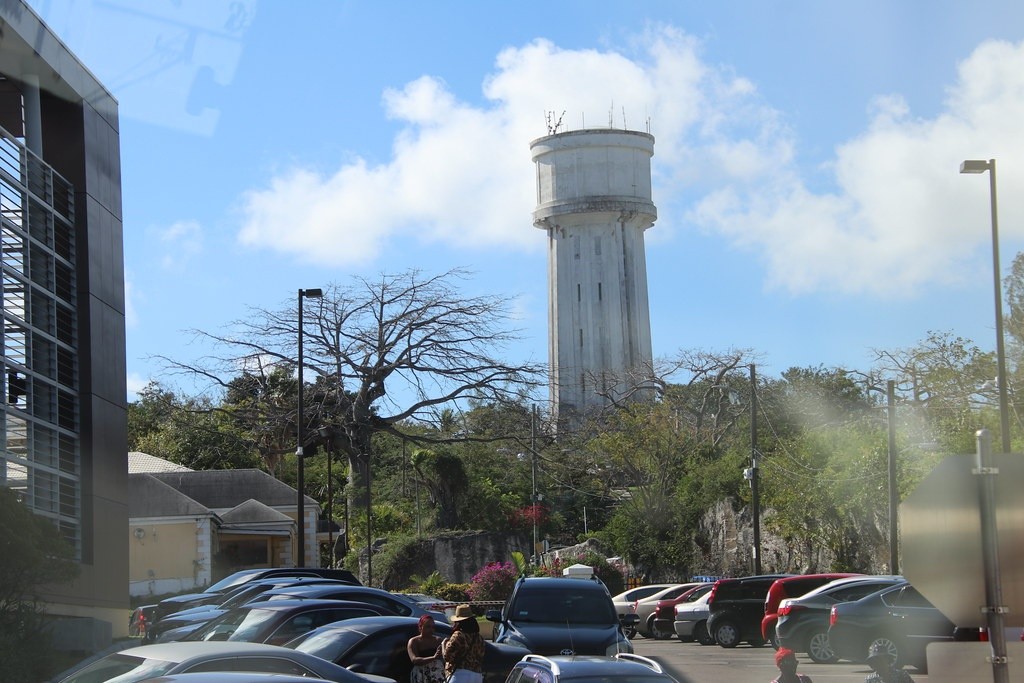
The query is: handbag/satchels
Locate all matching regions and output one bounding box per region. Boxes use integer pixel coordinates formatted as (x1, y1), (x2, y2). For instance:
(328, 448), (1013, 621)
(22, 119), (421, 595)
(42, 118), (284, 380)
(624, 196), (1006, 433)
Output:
(440, 677), (446, 683)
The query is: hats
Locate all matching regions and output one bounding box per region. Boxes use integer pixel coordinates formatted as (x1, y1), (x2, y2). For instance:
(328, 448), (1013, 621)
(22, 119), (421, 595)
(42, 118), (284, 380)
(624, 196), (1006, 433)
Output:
(450, 604), (476, 621)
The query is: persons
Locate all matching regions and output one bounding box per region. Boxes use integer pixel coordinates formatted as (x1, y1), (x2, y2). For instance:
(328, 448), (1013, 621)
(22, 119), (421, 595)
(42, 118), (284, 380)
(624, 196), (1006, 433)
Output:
(407, 615), (445, 683)
(441, 603), (486, 683)
(865, 646), (914, 683)
(769, 646), (813, 683)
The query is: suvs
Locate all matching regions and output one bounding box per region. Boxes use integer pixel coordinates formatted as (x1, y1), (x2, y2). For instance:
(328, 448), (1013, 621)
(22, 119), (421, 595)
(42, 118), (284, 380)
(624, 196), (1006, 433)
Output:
(485, 573), (641, 658)
(505, 652), (679, 683)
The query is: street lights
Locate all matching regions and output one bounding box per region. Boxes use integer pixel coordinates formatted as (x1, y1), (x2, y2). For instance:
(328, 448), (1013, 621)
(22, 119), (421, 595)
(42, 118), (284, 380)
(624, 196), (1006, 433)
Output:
(297, 288), (324, 568)
(960, 158), (1011, 457)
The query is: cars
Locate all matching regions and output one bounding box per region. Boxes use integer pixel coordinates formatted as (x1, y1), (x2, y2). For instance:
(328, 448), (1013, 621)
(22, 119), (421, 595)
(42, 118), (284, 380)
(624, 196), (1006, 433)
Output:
(46, 566), (532, 683)
(610, 572), (989, 672)
